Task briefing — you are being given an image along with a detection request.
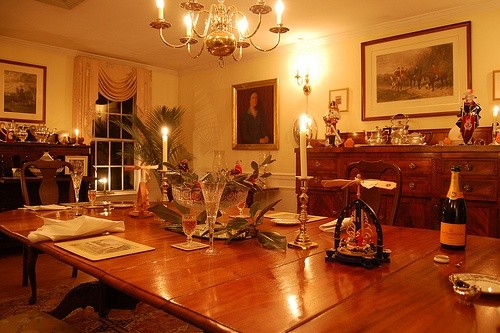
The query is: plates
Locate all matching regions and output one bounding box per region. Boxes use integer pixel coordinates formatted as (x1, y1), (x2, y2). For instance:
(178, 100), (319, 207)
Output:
(271, 218), (299, 224)
(448, 273), (500, 294)
(114, 204), (134, 207)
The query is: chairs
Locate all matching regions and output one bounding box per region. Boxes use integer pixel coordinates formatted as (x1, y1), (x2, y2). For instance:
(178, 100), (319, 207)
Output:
(20, 152), (78, 305)
(340, 159), (403, 225)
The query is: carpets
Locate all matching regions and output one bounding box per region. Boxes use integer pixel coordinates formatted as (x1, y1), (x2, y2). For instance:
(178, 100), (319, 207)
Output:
(0, 280), (204, 333)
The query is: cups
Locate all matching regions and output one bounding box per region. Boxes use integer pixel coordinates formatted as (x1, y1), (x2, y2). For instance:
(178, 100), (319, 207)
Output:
(69, 137), (77, 144)
(77, 138), (84, 144)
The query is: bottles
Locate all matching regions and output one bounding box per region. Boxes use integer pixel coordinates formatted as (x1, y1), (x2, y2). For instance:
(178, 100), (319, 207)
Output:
(440, 164), (467, 250)
(0, 119), (28, 143)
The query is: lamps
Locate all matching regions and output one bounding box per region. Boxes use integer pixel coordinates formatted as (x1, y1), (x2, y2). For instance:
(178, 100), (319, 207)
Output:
(295, 67), (312, 95)
(148, 0), (290, 68)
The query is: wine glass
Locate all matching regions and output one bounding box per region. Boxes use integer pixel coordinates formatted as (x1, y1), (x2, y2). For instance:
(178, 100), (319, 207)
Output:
(182, 213), (197, 248)
(70, 170), (84, 210)
(88, 189), (97, 208)
(198, 182), (226, 256)
(237, 202), (245, 218)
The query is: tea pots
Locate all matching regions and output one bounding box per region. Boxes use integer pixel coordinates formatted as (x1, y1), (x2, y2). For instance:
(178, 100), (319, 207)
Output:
(364, 126), (388, 145)
(389, 113), (410, 144)
(398, 130), (432, 146)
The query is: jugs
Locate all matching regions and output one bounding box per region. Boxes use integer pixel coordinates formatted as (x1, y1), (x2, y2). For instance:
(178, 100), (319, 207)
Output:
(29, 121), (58, 143)
(451, 274), (482, 306)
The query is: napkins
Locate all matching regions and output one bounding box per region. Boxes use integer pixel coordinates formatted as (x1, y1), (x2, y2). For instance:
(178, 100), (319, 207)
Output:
(28, 213), (125, 245)
(22, 204), (71, 211)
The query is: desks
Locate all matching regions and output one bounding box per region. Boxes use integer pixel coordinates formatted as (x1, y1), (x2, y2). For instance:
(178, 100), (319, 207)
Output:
(0, 138), (94, 206)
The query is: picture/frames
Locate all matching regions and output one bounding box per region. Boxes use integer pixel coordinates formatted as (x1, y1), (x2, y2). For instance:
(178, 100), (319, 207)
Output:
(493, 69), (500, 100)
(0, 58), (47, 124)
(64, 155), (88, 195)
(361, 20), (472, 121)
(231, 78), (280, 151)
(329, 88), (350, 112)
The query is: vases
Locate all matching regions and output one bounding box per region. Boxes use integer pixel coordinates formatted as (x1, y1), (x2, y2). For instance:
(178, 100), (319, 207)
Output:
(170, 185), (249, 239)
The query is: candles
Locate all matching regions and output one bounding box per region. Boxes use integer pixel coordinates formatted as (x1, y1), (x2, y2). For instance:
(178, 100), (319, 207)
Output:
(299, 113), (311, 176)
(492, 105), (500, 133)
(74, 129), (79, 143)
(161, 127), (170, 169)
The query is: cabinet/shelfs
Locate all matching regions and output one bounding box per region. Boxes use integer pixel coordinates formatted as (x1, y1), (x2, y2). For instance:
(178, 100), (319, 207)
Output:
(293, 142), (500, 241)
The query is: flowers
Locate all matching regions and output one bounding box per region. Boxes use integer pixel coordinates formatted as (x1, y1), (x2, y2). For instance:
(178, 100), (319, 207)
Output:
(160, 154), (288, 252)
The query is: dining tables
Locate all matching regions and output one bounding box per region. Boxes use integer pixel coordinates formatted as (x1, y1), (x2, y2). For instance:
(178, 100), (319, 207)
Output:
(0, 199), (500, 333)
